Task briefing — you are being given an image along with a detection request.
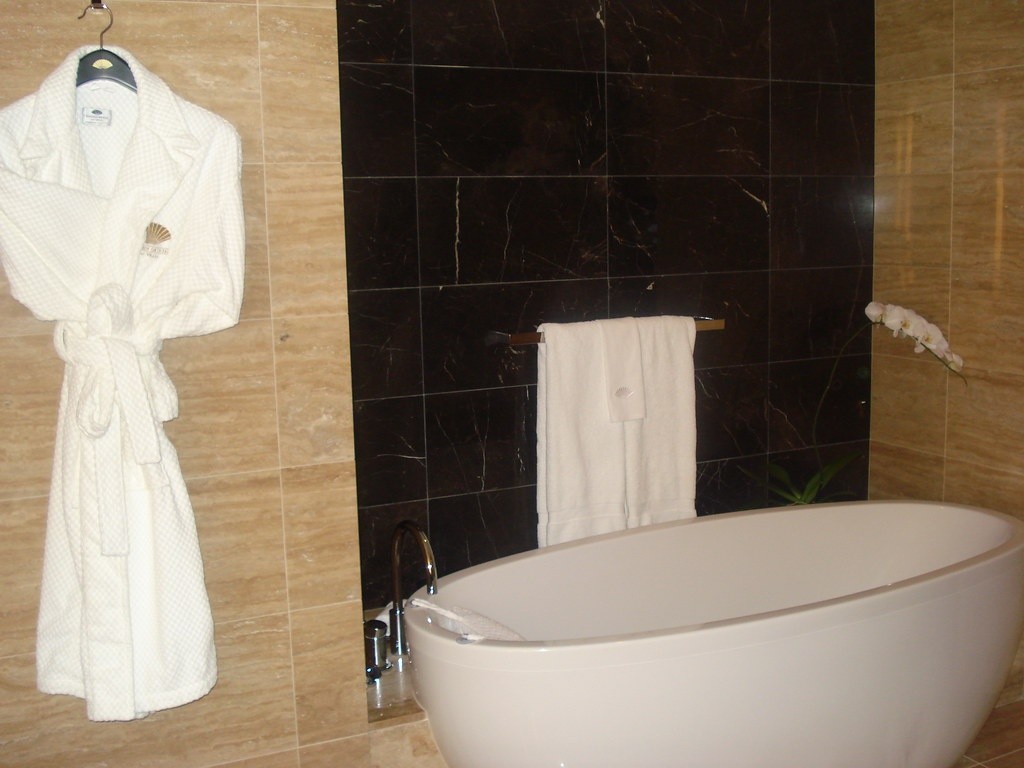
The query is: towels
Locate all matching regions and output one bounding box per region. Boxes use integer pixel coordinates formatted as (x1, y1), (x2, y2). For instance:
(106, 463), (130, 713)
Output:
(537, 315), (697, 550)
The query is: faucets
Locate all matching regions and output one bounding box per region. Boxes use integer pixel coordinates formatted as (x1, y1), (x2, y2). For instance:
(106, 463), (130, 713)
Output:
(387, 519), (438, 657)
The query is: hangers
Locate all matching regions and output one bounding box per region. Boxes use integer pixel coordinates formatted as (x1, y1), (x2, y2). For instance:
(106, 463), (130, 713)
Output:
(0, 3), (242, 153)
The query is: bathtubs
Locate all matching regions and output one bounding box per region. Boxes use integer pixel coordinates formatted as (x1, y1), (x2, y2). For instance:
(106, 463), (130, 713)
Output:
(400, 497), (1024, 767)
